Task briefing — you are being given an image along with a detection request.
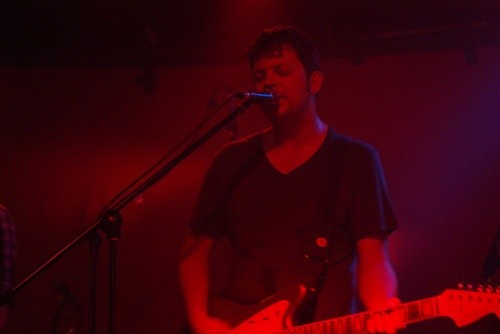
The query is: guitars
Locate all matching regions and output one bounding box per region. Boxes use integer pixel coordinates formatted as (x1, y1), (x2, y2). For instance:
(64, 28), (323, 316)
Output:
(210, 284), (500, 334)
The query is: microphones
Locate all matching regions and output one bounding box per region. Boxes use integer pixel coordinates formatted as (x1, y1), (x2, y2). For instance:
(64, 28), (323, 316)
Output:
(56, 283), (81, 309)
(235, 90), (278, 109)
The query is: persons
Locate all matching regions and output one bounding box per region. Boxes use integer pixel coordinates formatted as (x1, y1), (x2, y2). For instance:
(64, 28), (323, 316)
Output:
(177, 21), (409, 334)
